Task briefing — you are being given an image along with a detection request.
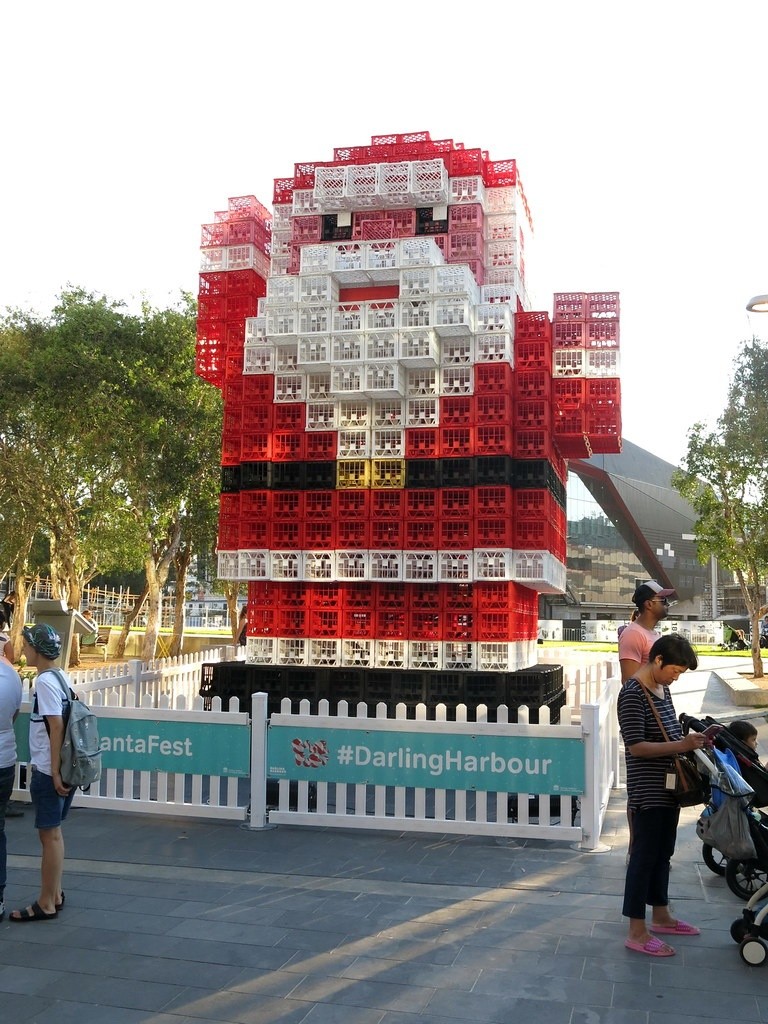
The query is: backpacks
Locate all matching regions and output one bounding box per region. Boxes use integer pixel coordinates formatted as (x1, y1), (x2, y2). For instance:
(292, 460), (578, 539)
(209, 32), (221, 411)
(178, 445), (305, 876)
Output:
(33, 669), (102, 792)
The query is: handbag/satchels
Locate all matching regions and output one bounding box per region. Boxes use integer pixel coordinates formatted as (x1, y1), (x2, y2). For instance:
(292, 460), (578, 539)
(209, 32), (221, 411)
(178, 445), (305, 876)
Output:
(671, 755), (706, 808)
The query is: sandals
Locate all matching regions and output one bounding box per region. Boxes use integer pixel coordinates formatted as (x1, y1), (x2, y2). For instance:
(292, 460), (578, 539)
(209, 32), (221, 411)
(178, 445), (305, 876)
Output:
(9, 900), (58, 922)
(55, 888), (65, 910)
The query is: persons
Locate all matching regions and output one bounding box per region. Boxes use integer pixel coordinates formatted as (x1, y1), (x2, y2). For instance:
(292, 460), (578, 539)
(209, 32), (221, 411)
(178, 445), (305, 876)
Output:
(9, 622), (78, 922)
(617, 633), (706, 957)
(0, 610), (14, 665)
(79, 610), (99, 649)
(618, 581), (675, 686)
(728, 720), (768, 772)
(0, 654), (22, 922)
(234, 604), (248, 646)
(761, 616), (768, 637)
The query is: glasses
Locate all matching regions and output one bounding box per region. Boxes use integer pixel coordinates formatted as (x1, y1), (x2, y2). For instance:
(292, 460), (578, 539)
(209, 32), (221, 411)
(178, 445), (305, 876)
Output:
(647, 598), (667, 605)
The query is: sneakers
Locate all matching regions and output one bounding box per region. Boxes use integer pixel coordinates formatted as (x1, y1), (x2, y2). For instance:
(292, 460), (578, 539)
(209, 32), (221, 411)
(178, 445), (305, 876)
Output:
(0, 902), (5, 915)
(625, 853), (672, 869)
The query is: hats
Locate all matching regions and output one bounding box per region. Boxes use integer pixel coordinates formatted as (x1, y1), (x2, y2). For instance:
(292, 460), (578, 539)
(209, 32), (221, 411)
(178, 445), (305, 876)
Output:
(21, 623), (62, 660)
(635, 581), (676, 607)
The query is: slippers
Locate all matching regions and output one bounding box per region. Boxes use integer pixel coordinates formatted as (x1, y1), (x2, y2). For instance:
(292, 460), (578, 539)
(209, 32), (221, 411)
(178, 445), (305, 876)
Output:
(623, 934), (675, 956)
(648, 919), (702, 934)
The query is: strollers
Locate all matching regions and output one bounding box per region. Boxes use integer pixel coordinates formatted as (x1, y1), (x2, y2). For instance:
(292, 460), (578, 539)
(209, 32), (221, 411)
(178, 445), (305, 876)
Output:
(723, 622), (751, 652)
(681, 713), (768, 899)
(728, 874), (768, 968)
